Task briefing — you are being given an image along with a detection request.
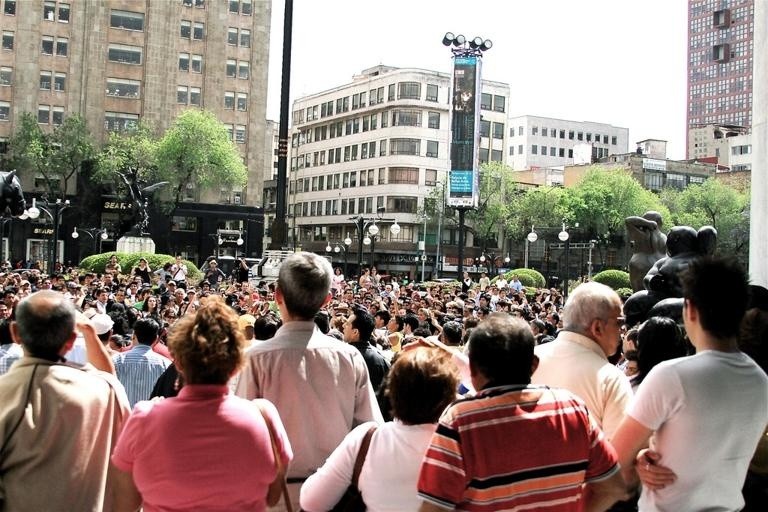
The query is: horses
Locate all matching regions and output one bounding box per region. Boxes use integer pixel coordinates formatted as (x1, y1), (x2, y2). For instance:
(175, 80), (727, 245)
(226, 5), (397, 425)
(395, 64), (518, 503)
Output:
(0, 167), (27, 218)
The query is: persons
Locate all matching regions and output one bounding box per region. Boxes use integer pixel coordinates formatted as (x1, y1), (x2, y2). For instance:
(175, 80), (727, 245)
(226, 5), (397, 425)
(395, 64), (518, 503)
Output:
(0, 252), (768, 512)
(123, 162), (142, 207)
(625, 211), (667, 292)
(624, 225), (717, 321)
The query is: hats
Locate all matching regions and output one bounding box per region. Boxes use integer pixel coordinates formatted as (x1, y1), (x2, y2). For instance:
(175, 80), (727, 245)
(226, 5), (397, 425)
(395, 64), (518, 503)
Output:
(82, 307), (98, 321)
(91, 313), (115, 336)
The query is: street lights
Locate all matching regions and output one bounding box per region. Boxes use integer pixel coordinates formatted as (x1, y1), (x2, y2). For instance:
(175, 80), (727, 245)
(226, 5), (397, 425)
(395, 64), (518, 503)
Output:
(29, 197), (71, 275)
(72, 226), (108, 254)
(557, 222), (576, 302)
(325, 215), (401, 290)
(1, 201), (29, 265)
(479, 250), (511, 280)
(527, 224), (538, 269)
(441, 31), (492, 280)
(420, 210), (431, 281)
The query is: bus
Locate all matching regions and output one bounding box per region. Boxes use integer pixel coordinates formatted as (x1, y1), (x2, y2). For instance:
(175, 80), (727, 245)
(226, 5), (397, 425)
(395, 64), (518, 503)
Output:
(200, 256), (263, 280)
